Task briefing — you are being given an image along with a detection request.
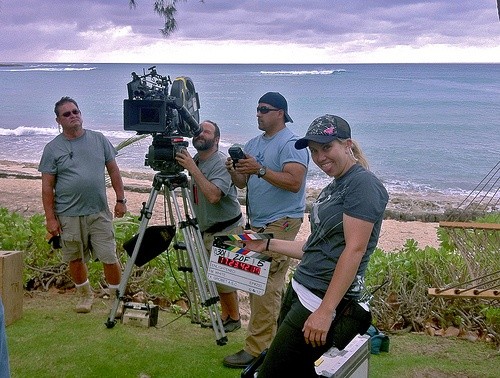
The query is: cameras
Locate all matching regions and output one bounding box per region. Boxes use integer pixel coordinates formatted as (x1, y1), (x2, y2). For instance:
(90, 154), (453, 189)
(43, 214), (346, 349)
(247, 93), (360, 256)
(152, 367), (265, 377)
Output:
(228, 147), (247, 165)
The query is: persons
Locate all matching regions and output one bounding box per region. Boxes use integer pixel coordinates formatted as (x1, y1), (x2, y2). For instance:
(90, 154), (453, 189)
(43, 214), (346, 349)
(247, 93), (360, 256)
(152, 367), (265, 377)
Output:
(238, 115), (389, 378)
(37, 95), (127, 318)
(225, 91), (310, 368)
(175, 119), (246, 333)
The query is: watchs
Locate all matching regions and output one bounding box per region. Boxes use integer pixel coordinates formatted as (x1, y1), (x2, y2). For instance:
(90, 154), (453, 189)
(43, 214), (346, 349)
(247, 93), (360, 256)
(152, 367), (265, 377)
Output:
(258, 165), (267, 178)
(115, 198), (127, 204)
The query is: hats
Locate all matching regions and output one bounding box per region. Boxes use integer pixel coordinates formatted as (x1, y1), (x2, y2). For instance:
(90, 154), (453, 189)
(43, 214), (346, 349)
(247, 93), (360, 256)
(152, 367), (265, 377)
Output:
(294, 114), (351, 150)
(259, 91), (293, 123)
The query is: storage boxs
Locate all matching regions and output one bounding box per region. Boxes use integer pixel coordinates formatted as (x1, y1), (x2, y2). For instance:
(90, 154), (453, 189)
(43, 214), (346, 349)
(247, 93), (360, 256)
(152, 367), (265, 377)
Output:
(314, 334), (371, 378)
(0, 251), (23, 328)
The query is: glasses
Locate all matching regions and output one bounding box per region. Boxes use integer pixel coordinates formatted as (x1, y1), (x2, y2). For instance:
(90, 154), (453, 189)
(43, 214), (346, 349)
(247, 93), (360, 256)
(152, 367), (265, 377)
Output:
(59, 108), (80, 117)
(257, 106), (278, 114)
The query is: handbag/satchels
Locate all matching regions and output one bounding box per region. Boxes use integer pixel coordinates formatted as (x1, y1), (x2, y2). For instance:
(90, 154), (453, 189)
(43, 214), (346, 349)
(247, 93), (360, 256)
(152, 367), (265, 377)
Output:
(367, 324), (391, 355)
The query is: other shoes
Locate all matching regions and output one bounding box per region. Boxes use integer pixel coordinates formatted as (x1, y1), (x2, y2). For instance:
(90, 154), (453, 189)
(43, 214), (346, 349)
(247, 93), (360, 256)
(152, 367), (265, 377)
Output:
(209, 318), (225, 329)
(223, 350), (256, 369)
(223, 316), (242, 333)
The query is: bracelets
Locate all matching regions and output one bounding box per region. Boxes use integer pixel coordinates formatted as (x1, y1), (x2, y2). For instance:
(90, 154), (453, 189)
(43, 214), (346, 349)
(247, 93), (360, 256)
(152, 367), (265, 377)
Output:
(265, 238), (271, 251)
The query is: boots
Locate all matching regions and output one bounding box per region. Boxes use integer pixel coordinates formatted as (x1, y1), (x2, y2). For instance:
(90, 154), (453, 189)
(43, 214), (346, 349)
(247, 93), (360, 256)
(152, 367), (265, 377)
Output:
(75, 281), (95, 313)
(108, 287), (124, 318)
